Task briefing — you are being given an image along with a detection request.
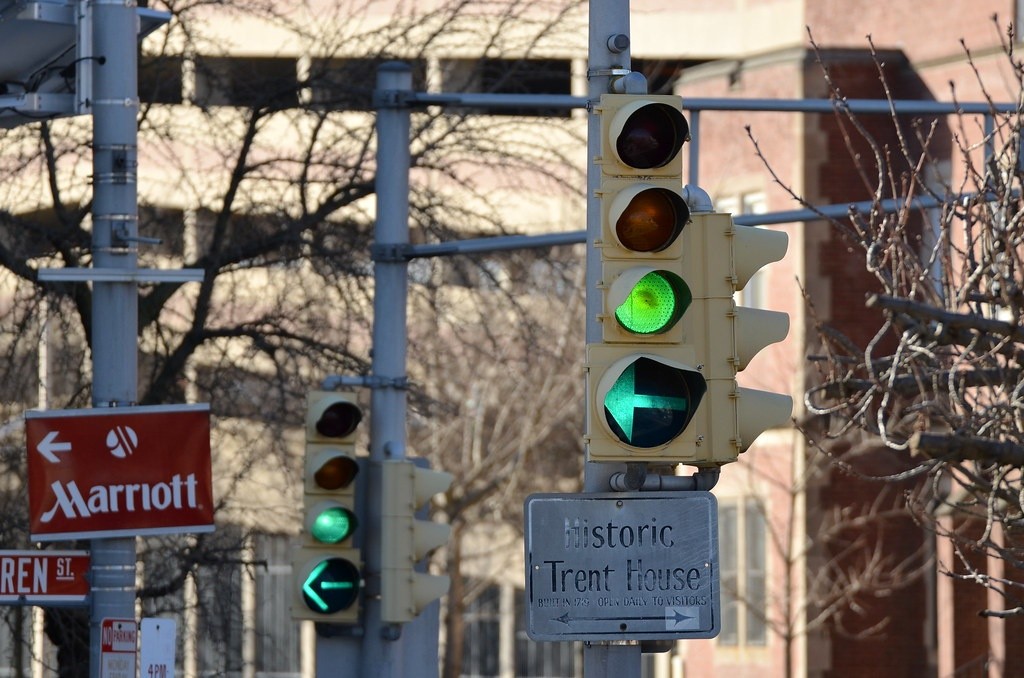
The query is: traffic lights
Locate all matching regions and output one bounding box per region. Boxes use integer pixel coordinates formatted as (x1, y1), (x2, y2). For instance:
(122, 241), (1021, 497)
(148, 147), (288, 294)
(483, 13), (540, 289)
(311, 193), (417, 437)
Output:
(301, 383), (362, 550)
(380, 458), (456, 625)
(292, 549), (361, 623)
(593, 90), (700, 349)
(582, 344), (713, 462)
(711, 220), (791, 465)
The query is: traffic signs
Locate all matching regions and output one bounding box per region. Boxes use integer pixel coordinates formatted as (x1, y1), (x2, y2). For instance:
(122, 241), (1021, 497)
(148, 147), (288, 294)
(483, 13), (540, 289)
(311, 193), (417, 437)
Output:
(18, 399), (217, 540)
